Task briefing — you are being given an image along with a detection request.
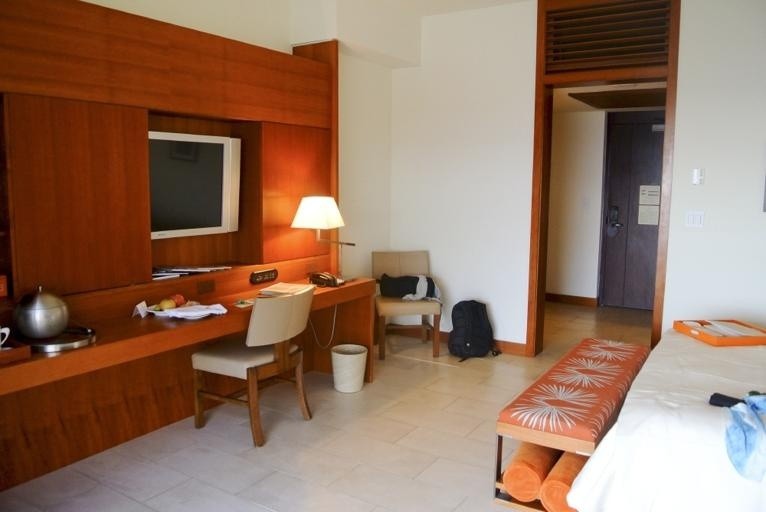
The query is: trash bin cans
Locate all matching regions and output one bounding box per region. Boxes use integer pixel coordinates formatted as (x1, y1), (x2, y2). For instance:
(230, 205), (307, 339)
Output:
(330, 344), (368, 394)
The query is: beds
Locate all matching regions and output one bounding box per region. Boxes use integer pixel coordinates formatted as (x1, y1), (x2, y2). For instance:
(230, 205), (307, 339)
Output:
(566, 323), (766, 512)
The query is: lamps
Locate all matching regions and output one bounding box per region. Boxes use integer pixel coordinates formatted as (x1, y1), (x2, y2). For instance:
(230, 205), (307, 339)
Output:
(289, 195), (358, 285)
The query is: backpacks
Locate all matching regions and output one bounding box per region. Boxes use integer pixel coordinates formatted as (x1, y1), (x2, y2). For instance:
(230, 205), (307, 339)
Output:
(448, 300), (502, 363)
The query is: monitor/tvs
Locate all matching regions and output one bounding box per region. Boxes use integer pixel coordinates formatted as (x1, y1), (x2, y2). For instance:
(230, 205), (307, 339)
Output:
(147, 130), (241, 241)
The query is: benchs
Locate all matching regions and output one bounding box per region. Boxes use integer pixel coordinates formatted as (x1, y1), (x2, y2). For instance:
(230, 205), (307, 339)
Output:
(493, 338), (650, 512)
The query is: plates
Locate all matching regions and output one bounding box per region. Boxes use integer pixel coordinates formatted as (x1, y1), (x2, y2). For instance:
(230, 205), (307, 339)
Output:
(146, 301), (200, 316)
(31, 336), (96, 352)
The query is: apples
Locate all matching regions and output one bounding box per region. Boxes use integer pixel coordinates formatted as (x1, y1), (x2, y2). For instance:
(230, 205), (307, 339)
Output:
(168, 294), (185, 307)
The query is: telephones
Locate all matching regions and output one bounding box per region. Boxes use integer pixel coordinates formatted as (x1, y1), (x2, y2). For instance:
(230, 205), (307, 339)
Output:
(309, 271), (345, 288)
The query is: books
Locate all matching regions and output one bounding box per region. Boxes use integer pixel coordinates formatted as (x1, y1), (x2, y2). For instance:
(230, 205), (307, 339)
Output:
(259, 282), (312, 295)
(150, 262), (232, 280)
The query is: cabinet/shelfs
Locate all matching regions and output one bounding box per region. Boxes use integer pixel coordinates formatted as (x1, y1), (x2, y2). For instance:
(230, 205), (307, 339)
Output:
(1, 253), (376, 492)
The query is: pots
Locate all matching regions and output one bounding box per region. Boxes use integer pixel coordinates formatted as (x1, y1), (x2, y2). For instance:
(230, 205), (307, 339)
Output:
(13, 284), (96, 336)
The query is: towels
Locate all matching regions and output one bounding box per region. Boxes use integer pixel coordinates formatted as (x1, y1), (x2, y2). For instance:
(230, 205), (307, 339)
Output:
(724, 391), (766, 483)
(166, 304), (227, 319)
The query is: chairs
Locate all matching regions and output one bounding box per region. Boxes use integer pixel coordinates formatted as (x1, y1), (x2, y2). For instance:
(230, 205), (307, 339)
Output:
(191, 285), (315, 448)
(372, 250), (442, 361)
